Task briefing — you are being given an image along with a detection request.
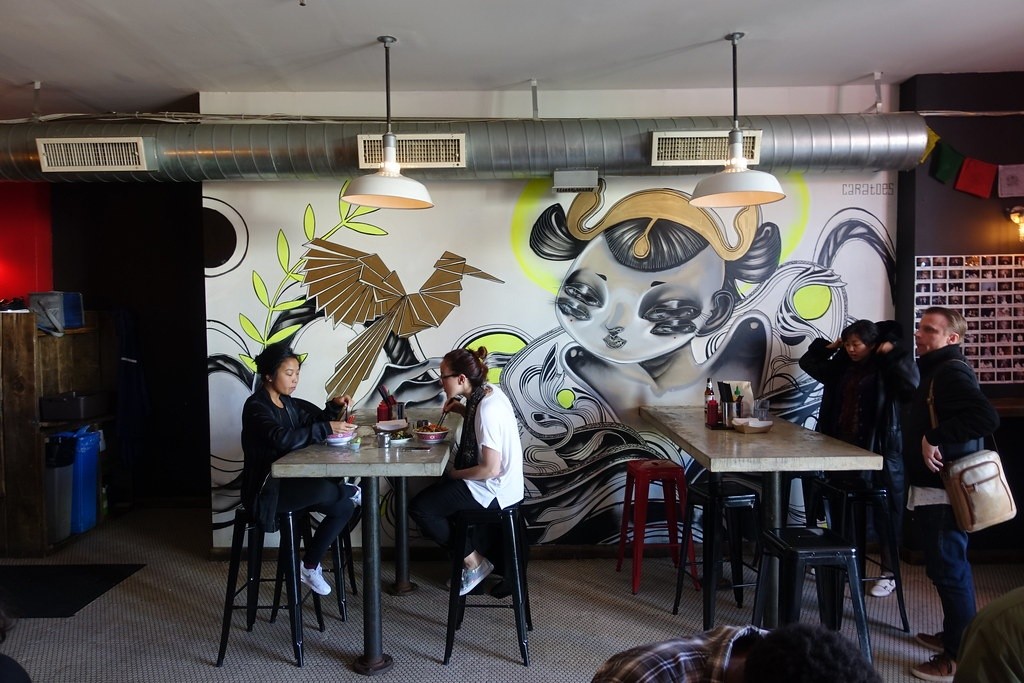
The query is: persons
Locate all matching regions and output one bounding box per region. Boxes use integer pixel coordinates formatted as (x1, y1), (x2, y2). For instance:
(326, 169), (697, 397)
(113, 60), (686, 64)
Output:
(799, 319), (920, 598)
(907, 307), (999, 681)
(916, 257), (1024, 381)
(241, 342), (362, 594)
(591, 624), (884, 683)
(406, 346), (524, 595)
(953, 586), (1024, 683)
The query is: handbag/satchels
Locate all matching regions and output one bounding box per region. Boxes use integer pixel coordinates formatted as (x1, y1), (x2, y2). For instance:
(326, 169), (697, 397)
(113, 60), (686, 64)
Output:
(945, 450), (1017, 533)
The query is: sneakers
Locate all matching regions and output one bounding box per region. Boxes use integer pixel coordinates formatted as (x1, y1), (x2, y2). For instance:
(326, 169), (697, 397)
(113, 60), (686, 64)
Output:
(910, 652), (957, 682)
(300, 560), (332, 595)
(459, 557), (494, 596)
(917, 633), (945, 653)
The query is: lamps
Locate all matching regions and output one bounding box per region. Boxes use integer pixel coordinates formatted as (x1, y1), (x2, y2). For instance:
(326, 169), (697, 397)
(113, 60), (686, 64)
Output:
(339, 35), (435, 210)
(688, 32), (786, 209)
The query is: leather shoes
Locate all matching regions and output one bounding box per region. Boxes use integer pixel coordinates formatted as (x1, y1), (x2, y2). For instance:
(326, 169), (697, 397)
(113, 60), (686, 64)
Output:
(870, 576), (896, 597)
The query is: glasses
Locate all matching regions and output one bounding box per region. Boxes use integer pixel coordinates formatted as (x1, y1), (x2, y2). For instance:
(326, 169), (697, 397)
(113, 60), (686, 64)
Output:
(440, 373), (456, 382)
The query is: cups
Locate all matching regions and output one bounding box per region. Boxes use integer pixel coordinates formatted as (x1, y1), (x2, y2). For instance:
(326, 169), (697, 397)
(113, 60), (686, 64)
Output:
(377, 432), (392, 447)
(723, 402), (743, 427)
(754, 399), (769, 420)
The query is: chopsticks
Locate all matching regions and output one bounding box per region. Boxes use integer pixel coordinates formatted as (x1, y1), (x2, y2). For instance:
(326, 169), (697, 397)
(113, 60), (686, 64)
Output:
(435, 396), (455, 428)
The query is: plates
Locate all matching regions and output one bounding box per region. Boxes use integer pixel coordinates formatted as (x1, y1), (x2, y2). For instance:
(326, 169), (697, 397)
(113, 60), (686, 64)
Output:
(391, 434), (414, 443)
(373, 426), (409, 433)
(325, 437), (354, 445)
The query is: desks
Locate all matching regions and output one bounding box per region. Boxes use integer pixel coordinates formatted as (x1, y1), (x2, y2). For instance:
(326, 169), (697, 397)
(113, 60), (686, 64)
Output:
(271, 409), (452, 677)
(639, 403), (883, 634)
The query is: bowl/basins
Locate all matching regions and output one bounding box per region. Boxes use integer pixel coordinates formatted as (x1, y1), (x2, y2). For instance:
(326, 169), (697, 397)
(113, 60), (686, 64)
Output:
(413, 427), (451, 443)
(348, 442), (360, 449)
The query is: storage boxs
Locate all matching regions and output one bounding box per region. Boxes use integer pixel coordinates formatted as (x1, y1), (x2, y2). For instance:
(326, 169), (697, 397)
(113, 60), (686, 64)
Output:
(38, 390), (118, 545)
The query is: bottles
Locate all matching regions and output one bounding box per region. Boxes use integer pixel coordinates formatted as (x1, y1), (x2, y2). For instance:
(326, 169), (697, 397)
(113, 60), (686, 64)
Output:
(704, 377), (714, 413)
(377, 390), (397, 421)
(733, 385), (741, 401)
(707, 397), (718, 426)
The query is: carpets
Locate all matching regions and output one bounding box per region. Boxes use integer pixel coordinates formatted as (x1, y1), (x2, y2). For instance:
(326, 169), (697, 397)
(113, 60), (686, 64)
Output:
(0, 564), (148, 619)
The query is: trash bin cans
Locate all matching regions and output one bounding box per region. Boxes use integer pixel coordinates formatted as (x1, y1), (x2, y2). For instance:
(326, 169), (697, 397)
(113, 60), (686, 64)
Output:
(44, 436), (76, 544)
(52, 425), (102, 537)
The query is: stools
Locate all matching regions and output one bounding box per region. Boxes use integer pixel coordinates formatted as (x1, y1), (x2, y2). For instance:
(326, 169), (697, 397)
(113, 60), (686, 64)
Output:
(215, 500), (360, 666)
(618, 460), (911, 664)
(442, 504), (534, 668)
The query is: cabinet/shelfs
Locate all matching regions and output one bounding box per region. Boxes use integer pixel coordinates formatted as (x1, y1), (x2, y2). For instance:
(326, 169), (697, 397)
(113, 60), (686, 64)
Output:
(0, 308), (125, 559)
